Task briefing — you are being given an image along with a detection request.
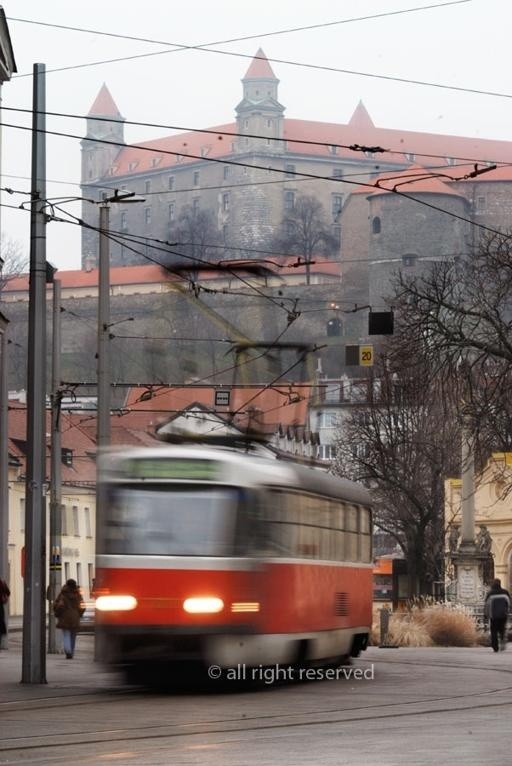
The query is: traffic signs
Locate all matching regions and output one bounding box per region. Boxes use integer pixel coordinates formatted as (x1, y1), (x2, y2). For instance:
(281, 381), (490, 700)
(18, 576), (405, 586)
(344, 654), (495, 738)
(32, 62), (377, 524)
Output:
(358, 345), (374, 366)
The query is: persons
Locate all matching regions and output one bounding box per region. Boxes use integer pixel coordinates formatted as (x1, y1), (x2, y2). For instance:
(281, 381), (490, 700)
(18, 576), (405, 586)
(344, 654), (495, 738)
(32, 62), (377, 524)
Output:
(449, 524), (461, 553)
(484, 579), (510, 652)
(0, 578), (10, 643)
(477, 524), (492, 552)
(380, 602), (391, 647)
(55, 578), (86, 658)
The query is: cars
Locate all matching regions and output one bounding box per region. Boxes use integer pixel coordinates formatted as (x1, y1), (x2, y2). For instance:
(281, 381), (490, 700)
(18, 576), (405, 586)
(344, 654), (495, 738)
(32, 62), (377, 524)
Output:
(78, 604), (94, 633)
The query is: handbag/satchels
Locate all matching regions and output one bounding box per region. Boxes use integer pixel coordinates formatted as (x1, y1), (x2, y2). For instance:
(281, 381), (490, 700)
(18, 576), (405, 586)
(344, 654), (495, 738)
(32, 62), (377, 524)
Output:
(0, 581), (7, 603)
(54, 592), (64, 616)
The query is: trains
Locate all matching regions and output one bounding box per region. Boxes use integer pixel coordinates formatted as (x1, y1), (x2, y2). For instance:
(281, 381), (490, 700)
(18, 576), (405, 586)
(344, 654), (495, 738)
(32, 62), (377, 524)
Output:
(95, 439), (374, 693)
